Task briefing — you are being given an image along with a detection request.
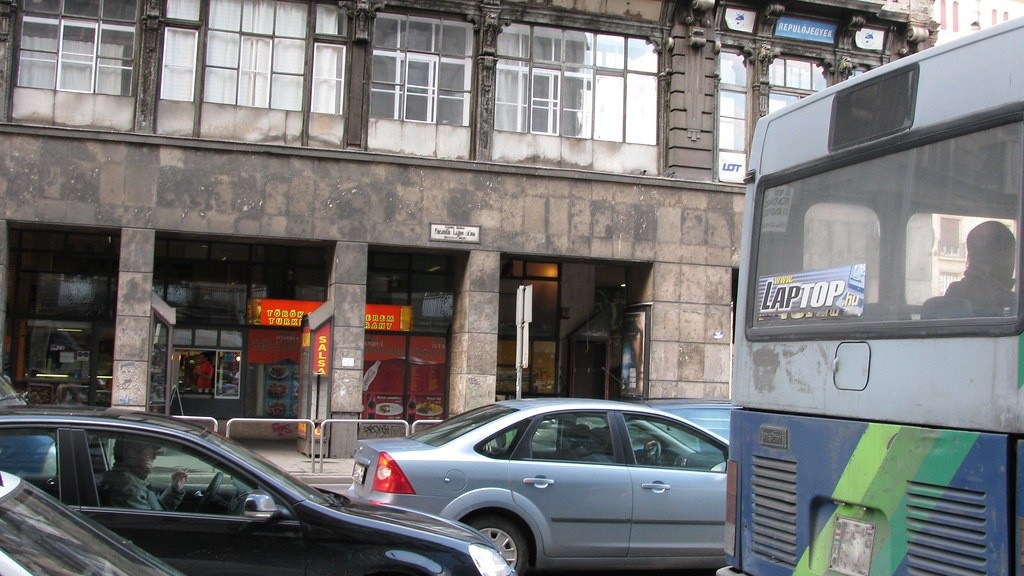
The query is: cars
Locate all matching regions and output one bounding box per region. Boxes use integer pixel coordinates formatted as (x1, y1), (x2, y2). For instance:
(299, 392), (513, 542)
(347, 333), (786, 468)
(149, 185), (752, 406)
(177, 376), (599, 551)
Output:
(0, 471), (185, 575)
(0, 399), (518, 576)
(343, 397), (731, 576)
(513, 395), (731, 461)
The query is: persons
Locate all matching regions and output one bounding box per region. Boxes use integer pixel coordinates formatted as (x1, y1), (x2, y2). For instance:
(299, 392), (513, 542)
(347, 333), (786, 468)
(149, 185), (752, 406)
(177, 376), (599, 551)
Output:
(624, 312), (644, 395)
(100, 440), (189, 511)
(603, 426), (656, 458)
(194, 352), (213, 393)
(942, 217), (1018, 317)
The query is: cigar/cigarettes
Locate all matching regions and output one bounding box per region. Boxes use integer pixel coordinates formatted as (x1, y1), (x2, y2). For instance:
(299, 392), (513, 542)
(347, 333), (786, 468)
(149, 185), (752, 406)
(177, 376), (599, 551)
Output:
(184, 467), (189, 473)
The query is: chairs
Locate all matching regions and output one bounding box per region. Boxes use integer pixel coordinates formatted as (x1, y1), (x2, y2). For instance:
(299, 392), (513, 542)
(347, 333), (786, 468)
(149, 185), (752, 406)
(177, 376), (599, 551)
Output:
(569, 424), (589, 461)
(920, 296), (975, 319)
(589, 427), (613, 463)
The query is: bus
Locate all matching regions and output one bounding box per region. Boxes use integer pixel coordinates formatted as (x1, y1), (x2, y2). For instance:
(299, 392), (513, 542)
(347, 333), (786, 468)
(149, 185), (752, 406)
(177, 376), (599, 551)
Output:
(715, 14), (1023, 575)
